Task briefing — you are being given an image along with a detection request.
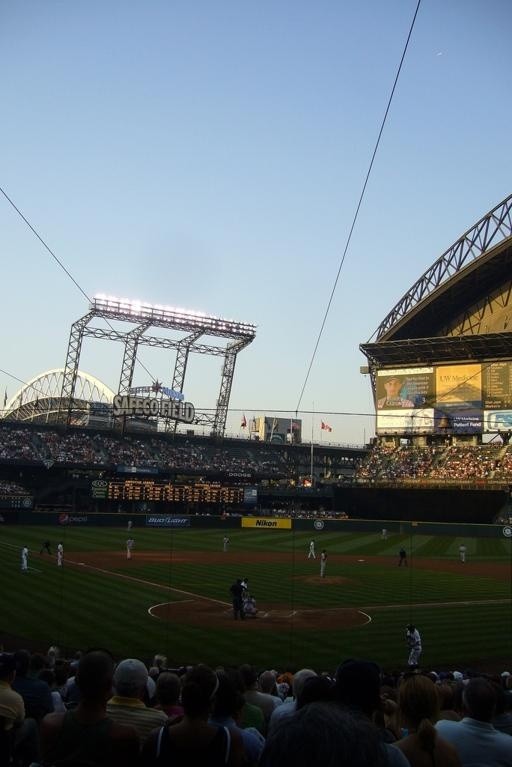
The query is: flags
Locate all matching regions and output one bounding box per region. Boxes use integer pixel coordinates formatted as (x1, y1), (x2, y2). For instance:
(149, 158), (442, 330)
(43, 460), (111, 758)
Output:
(292, 420), (299, 429)
(5, 389), (71, 414)
(322, 422), (331, 431)
(253, 415), (257, 429)
(241, 415), (248, 427)
(273, 416), (279, 430)
(264, 416), (269, 425)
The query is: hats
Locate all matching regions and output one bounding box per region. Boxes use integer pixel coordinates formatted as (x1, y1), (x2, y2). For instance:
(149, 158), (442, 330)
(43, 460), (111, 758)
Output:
(292, 668), (318, 696)
(114, 658), (149, 694)
(259, 670), (276, 689)
(335, 658), (382, 707)
(462, 677), (497, 722)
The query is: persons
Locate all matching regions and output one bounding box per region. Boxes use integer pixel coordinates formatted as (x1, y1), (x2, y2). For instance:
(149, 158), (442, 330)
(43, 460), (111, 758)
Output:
(349, 441), (511, 495)
(1, 426), (348, 525)
(127, 520), (134, 530)
(377, 374), (415, 408)
(230, 576), (259, 620)
(39, 535), (53, 555)
(126, 535), (137, 560)
(458, 543), (467, 564)
(495, 514), (511, 526)
(382, 527), (389, 541)
(21, 545), (30, 570)
(398, 546), (408, 568)
(404, 623), (425, 665)
(307, 538), (317, 560)
(57, 540), (65, 567)
(223, 535), (230, 552)
(1, 639), (511, 767)
(320, 549), (329, 578)
